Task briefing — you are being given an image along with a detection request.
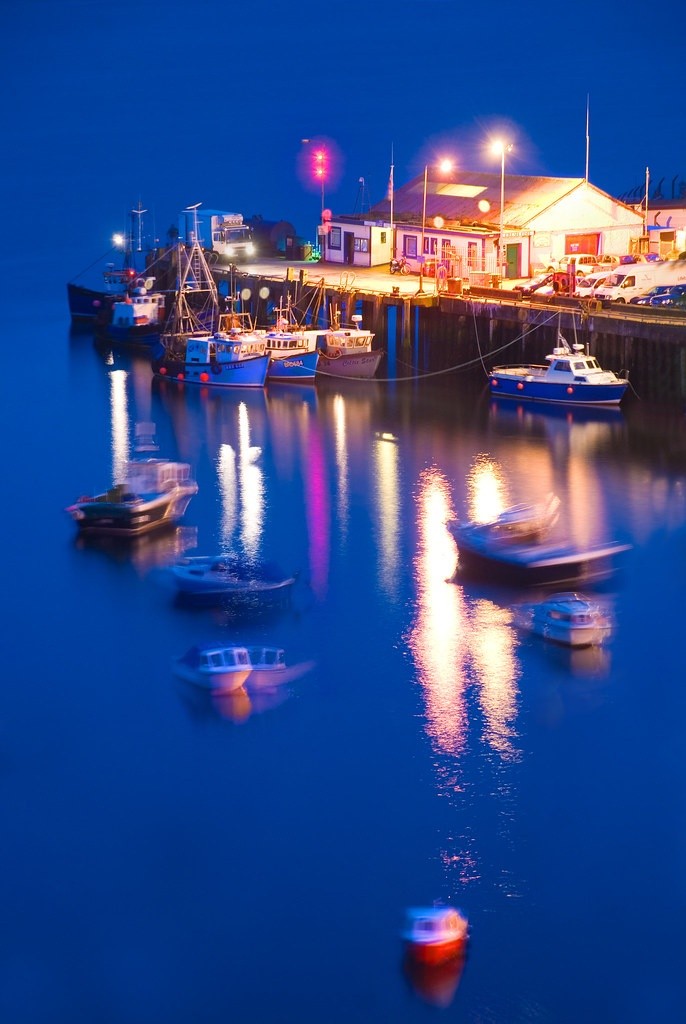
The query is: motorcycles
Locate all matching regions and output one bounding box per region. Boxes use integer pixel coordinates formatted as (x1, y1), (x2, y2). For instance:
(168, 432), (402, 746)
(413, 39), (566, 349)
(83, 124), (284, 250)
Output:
(389, 253), (411, 276)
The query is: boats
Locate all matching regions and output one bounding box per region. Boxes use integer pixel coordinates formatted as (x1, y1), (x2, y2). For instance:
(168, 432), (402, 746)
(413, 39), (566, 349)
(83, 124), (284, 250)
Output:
(67, 457), (199, 538)
(66, 201), (385, 381)
(444, 493), (634, 592)
(488, 305), (630, 406)
(399, 905), (472, 965)
(146, 202), (271, 388)
(186, 646), (253, 695)
(508, 591), (612, 648)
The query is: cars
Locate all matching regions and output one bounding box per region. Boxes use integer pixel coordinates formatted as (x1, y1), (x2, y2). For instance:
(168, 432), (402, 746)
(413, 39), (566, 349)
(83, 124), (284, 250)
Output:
(512, 251), (686, 308)
(650, 283), (686, 308)
(630, 285), (673, 306)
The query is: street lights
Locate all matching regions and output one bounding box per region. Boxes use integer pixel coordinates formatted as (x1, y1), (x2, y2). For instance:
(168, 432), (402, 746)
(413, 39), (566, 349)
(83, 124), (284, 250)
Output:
(490, 141), (505, 290)
(416, 161), (451, 296)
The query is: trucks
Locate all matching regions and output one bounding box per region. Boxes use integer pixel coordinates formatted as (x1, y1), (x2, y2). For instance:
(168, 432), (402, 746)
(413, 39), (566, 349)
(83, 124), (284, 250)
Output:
(177, 209), (256, 266)
